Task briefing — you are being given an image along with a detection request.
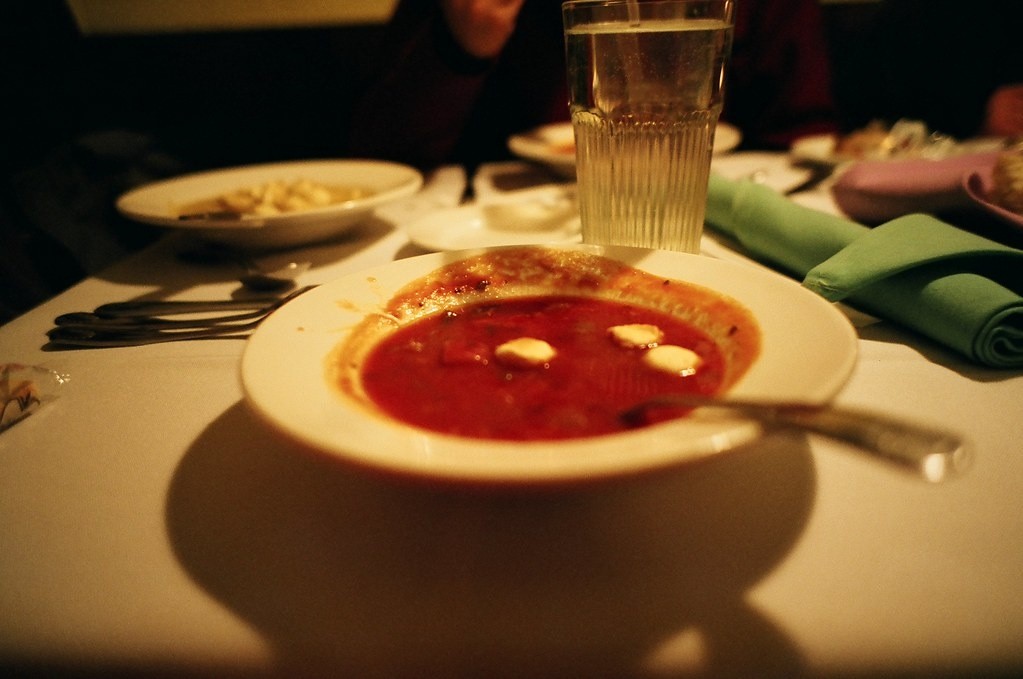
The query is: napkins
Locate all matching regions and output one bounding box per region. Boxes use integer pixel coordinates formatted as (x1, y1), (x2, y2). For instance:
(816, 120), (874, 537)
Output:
(704, 169), (1023, 371)
(798, 212), (1023, 305)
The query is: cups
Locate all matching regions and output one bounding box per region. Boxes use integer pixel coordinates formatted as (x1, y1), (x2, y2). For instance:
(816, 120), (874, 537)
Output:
(560, 0), (735, 255)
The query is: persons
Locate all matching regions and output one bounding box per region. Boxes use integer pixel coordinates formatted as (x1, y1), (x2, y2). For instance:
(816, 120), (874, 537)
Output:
(374, 0), (598, 172)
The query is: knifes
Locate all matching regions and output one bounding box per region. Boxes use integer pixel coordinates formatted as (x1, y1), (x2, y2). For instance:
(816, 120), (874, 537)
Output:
(47, 283), (318, 346)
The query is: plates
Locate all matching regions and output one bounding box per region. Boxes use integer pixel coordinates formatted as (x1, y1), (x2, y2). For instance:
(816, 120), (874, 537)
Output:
(239, 243), (861, 488)
(116, 157), (424, 251)
(507, 120), (742, 177)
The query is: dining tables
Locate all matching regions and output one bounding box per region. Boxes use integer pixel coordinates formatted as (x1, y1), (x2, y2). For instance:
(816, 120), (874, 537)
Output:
(1, 152), (1023, 679)
